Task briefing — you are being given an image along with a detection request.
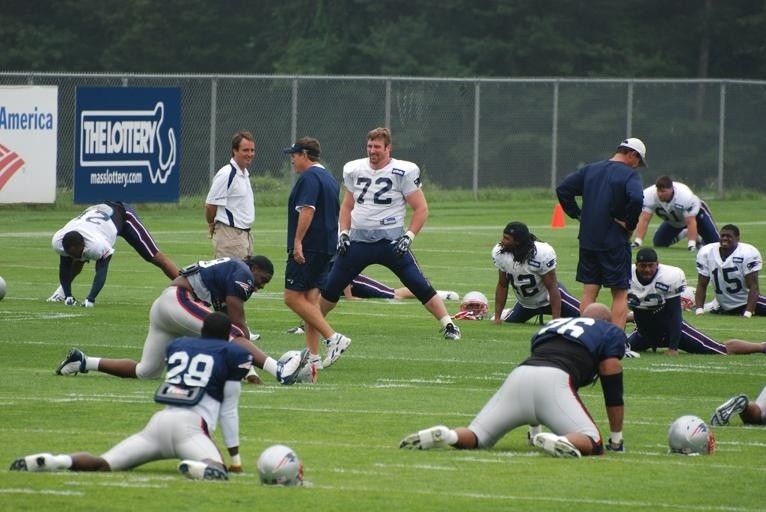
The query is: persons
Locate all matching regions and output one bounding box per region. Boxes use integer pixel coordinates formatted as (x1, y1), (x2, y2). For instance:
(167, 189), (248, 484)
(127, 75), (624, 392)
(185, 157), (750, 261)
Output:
(555, 137), (648, 357)
(631, 174), (721, 253)
(708, 383), (766, 427)
(284, 126), (463, 342)
(283, 135), (351, 378)
(692, 224), (765, 317)
(203, 128), (262, 344)
(342, 275), (461, 305)
(395, 302), (629, 459)
(53, 256), (311, 390)
(6, 311), (242, 484)
(45, 200), (179, 307)
(488, 221), (580, 324)
(622, 246), (765, 359)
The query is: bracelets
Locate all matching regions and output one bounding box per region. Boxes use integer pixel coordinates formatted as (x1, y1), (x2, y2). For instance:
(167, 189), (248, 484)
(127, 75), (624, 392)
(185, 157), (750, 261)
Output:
(227, 452), (241, 468)
(609, 429), (624, 446)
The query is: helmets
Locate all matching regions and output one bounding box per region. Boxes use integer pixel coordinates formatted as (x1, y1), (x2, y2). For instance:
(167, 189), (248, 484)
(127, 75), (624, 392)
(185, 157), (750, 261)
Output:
(680, 285), (698, 311)
(277, 350), (311, 383)
(666, 413), (718, 457)
(254, 444), (302, 487)
(460, 289), (489, 314)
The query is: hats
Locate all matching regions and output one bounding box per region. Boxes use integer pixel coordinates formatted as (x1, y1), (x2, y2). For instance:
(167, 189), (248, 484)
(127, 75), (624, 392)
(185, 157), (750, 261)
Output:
(636, 246), (658, 264)
(617, 136), (652, 169)
(282, 134), (323, 158)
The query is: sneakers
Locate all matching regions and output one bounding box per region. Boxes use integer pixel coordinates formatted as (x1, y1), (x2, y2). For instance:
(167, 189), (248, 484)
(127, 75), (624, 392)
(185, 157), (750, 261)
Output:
(709, 391), (749, 427)
(442, 322), (462, 341)
(531, 431), (582, 458)
(624, 350), (642, 360)
(275, 347), (311, 386)
(435, 288), (460, 304)
(8, 451), (54, 473)
(55, 345), (89, 377)
(309, 350), (324, 370)
(397, 424), (452, 451)
(45, 293), (65, 304)
(175, 459), (229, 482)
(320, 329), (352, 371)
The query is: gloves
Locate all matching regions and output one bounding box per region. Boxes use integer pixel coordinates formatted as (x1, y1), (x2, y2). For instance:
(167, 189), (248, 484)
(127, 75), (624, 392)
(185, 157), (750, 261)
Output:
(389, 231), (416, 257)
(79, 298), (94, 309)
(335, 232), (351, 256)
(631, 240), (641, 249)
(64, 295), (77, 306)
(687, 245), (697, 253)
